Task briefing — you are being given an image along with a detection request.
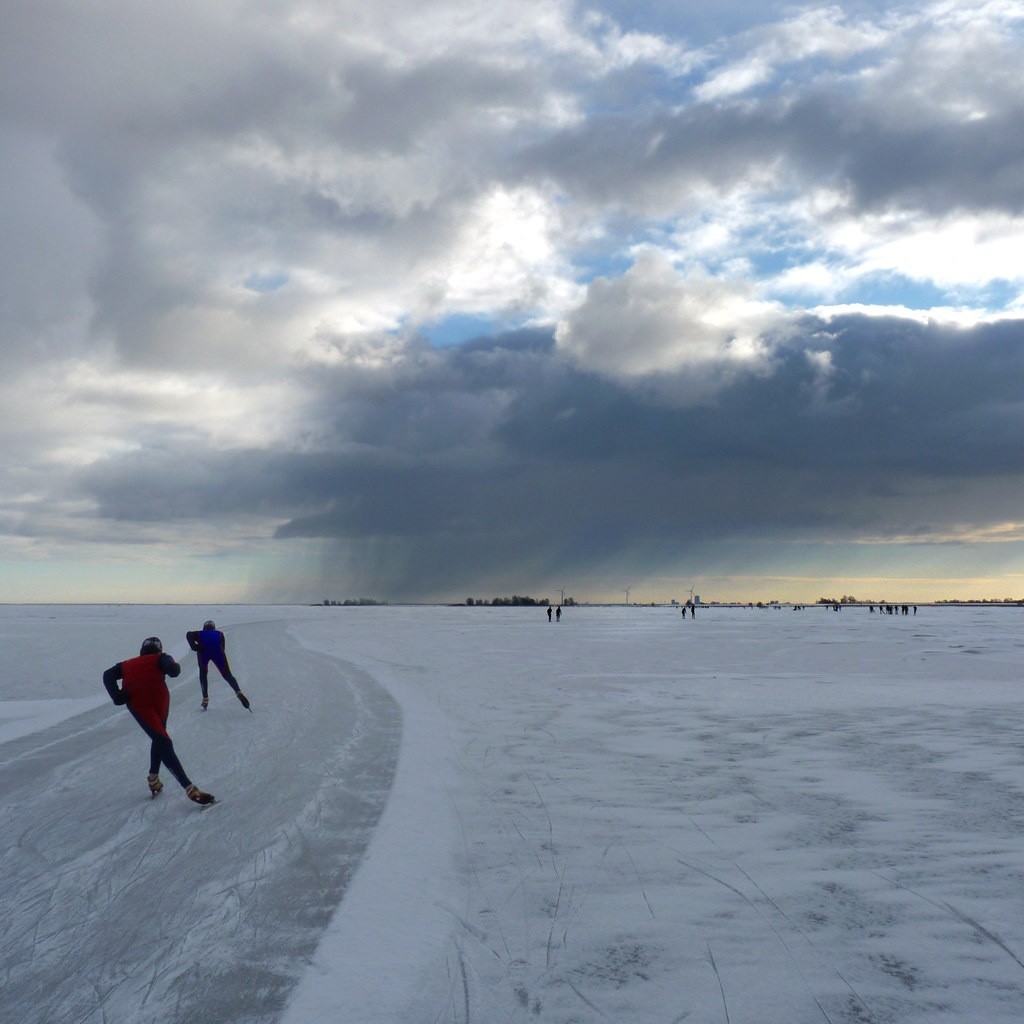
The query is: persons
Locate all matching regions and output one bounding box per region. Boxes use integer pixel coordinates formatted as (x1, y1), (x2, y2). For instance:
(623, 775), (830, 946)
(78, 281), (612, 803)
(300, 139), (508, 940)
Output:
(103, 637), (220, 809)
(547, 607), (552, 622)
(556, 606), (561, 622)
(682, 607), (686, 619)
(187, 621), (252, 712)
(691, 604), (695, 619)
(795, 606), (805, 610)
(826, 604), (841, 611)
(869, 604), (917, 616)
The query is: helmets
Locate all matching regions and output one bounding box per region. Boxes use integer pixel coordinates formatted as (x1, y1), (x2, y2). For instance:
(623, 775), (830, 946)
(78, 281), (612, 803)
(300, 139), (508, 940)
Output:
(204, 620), (215, 628)
(142, 637), (162, 651)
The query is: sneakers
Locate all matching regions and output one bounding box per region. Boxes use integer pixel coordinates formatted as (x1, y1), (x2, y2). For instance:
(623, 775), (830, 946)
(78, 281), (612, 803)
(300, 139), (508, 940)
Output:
(146, 776), (163, 800)
(200, 699), (209, 710)
(187, 786), (221, 814)
(239, 695), (252, 712)
